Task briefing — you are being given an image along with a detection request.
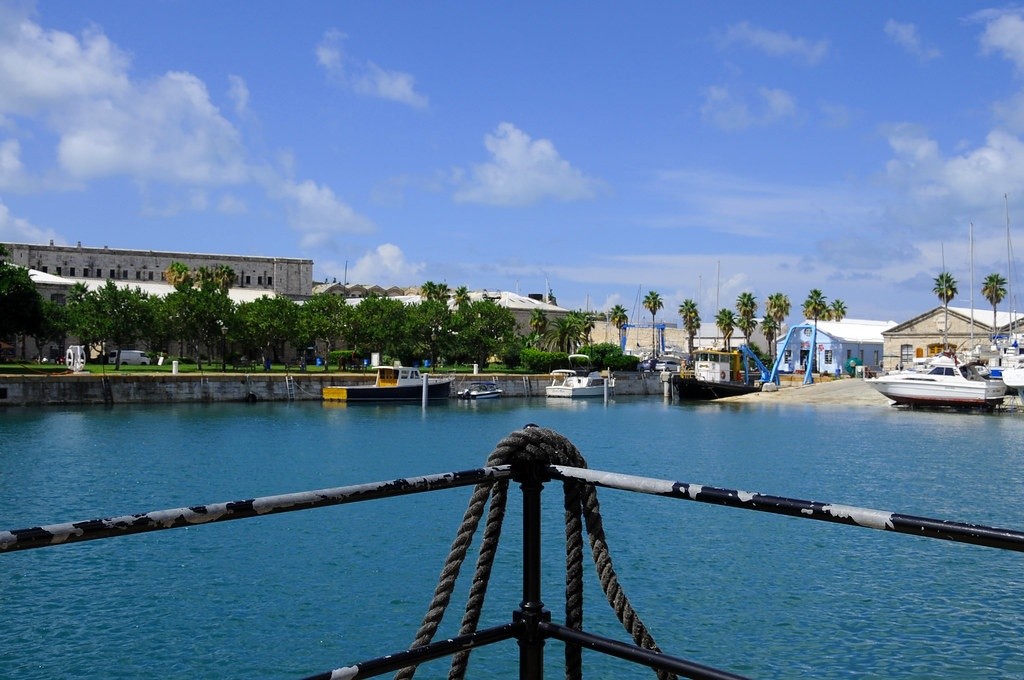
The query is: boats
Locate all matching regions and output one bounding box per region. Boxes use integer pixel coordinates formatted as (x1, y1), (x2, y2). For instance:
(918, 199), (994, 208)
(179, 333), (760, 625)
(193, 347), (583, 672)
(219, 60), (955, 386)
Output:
(456, 380), (504, 400)
(672, 373), (761, 399)
(544, 369), (614, 399)
(322, 366), (455, 402)
(864, 354), (1007, 404)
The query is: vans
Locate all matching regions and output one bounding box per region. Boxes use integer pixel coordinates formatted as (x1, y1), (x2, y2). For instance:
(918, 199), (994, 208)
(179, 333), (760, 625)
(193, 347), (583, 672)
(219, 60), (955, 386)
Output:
(109, 350), (152, 365)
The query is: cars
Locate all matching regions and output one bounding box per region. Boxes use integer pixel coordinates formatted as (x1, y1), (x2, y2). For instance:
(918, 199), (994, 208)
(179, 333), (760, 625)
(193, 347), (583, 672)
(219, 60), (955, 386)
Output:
(655, 360), (679, 371)
